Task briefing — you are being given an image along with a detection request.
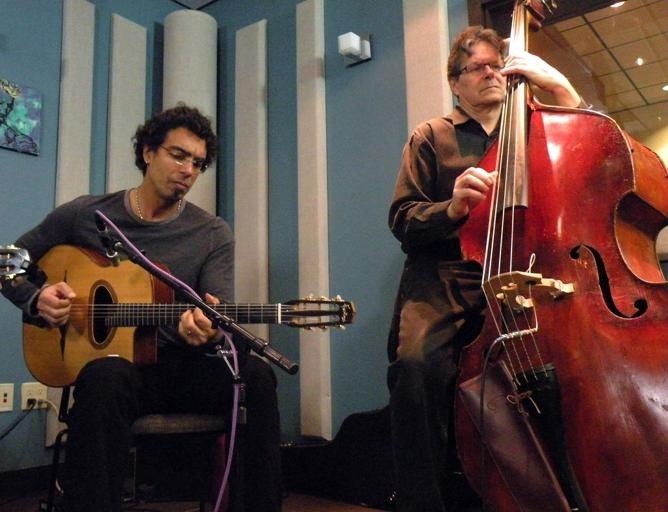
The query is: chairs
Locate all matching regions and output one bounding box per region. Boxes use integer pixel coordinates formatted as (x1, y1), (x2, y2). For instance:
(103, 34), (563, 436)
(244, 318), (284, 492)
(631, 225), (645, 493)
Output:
(47, 388), (247, 511)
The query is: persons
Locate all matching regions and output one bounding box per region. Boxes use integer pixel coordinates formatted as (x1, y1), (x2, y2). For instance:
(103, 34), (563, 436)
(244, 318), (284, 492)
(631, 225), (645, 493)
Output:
(386, 23), (594, 511)
(0, 104), (280, 510)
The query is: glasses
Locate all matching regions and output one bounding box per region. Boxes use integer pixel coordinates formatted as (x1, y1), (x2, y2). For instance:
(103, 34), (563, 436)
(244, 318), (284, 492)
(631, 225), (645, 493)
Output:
(158, 143), (208, 172)
(459, 62), (505, 74)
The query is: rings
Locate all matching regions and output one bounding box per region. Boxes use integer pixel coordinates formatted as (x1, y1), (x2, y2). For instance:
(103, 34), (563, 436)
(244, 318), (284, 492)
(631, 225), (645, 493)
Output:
(185, 330), (191, 336)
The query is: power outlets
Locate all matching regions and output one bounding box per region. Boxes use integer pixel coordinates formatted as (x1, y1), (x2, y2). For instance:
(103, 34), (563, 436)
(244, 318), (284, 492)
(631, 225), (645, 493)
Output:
(21, 383), (48, 409)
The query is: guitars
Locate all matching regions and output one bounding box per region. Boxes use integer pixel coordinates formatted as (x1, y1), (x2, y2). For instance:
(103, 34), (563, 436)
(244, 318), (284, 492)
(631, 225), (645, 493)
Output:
(23, 244), (359, 388)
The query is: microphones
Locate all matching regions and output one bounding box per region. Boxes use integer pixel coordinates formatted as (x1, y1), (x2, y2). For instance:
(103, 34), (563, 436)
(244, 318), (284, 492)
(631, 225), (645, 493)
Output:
(92, 211), (121, 268)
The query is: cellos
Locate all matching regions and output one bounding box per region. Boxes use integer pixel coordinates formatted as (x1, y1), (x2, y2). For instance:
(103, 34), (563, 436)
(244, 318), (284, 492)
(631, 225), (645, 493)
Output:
(453, 0), (667, 512)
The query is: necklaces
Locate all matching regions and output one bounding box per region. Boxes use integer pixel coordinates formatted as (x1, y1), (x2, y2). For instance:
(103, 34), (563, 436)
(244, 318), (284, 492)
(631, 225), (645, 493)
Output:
(135, 186), (182, 220)
